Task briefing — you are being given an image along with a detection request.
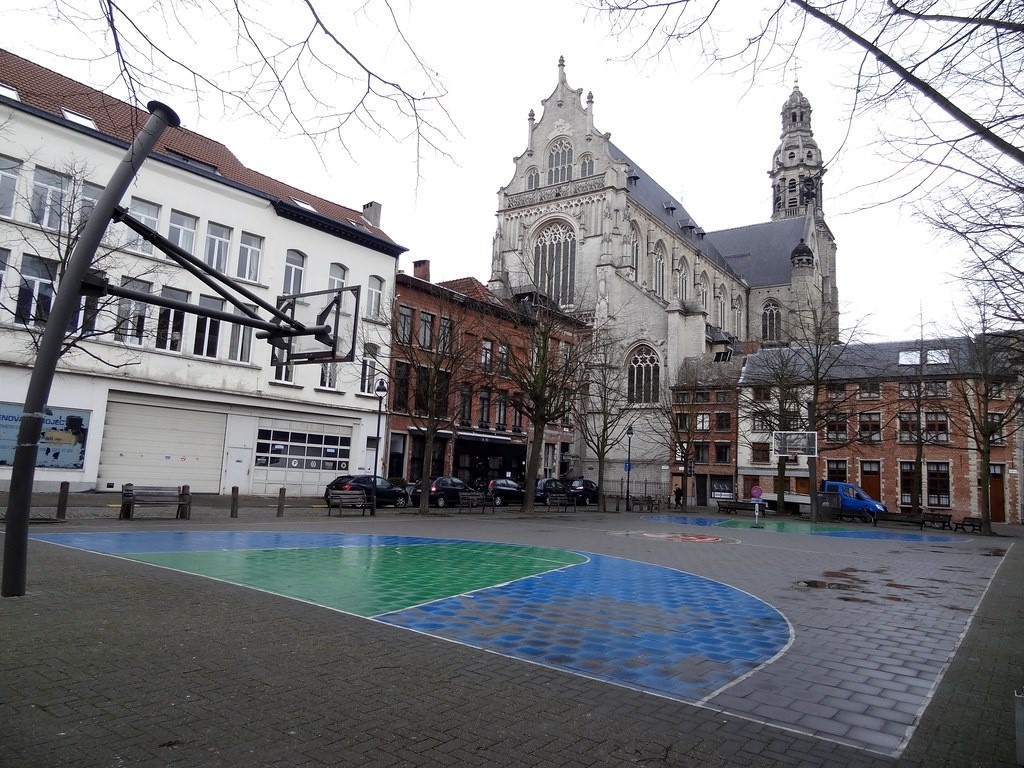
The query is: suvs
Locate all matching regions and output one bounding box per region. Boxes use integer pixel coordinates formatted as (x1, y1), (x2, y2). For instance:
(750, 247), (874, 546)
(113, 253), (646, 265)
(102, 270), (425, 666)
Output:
(485, 479), (526, 507)
(566, 480), (598, 506)
(411, 474), (478, 507)
(324, 475), (408, 509)
(523, 477), (571, 506)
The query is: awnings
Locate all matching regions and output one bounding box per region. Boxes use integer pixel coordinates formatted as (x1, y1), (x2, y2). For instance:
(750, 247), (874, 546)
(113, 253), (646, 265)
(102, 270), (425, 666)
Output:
(408, 427), (511, 445)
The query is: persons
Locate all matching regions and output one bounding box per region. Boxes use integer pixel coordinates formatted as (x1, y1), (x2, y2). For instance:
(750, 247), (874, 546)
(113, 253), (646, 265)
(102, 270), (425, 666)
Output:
(673, 486), (682, 509)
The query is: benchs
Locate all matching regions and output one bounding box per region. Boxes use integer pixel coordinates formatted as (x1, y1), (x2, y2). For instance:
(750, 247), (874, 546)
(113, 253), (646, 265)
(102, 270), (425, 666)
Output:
(329, 490), (377, 517)
(120, 484), (194, 521)
(716, 500), (767, 518)
(822, 505), (874, 523)
(954, 516), (982, 533)
(630, 494), (661, 514)
(873, 509), (924, 530)
(547, 495), (577, 513)
(921, 512), (953, 532)
(459, 492), (495, 514)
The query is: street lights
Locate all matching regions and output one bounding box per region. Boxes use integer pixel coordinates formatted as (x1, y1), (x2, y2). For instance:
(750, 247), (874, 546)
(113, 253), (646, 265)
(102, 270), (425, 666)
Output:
(624, 425), (634, 512)
(370, 378), (390, 516)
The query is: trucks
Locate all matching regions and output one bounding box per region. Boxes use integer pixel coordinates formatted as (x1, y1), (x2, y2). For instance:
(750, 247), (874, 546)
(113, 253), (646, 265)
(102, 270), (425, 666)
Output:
(760, 479), (887, 523)
